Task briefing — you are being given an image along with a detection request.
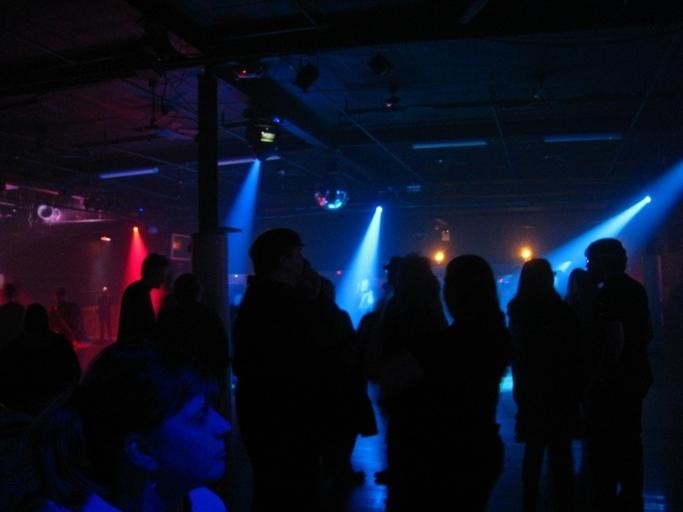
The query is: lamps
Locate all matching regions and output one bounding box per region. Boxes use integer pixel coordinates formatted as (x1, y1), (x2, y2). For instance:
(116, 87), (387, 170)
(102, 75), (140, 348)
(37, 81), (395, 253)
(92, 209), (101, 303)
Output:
(241, 104), (282, 162)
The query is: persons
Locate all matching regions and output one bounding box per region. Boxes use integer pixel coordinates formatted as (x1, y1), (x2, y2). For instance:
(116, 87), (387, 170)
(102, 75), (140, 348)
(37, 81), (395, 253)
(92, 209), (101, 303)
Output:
(2, 226), (657, 511)
